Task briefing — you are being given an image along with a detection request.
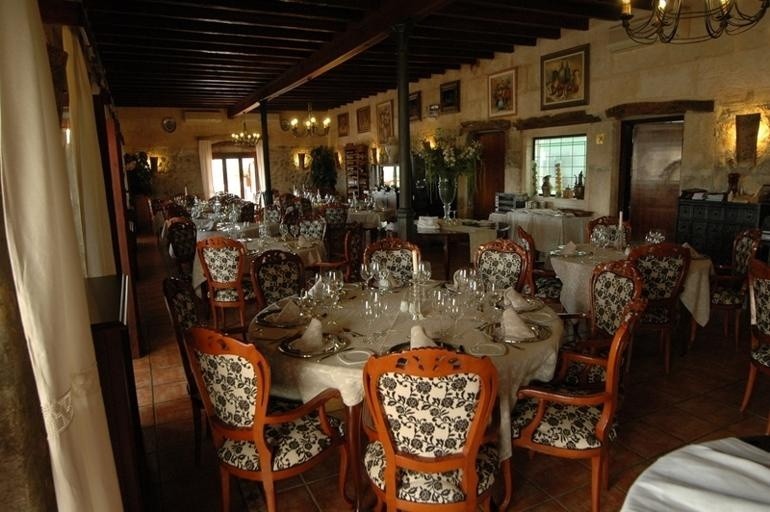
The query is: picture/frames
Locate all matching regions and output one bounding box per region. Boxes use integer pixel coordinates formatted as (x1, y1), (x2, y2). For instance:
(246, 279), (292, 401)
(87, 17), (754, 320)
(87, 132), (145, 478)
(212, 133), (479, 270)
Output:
(338, 80), (463, 150)
(486, 39), (593, 119)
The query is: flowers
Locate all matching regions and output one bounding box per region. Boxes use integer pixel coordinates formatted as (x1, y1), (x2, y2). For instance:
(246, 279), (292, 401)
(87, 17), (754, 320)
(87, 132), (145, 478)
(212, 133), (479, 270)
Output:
(409, 125), (485, 186)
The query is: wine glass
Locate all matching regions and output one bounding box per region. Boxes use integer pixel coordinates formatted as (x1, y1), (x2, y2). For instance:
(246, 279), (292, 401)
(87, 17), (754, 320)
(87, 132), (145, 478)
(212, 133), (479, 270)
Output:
(309, 259), (502, 349)
(451, 211), (458, 225)
(294, 184), (375, 213)
(192, 197), (313, 253)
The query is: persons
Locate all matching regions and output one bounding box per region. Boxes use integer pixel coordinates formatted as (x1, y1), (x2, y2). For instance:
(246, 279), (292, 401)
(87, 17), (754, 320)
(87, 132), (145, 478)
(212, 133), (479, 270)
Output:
(159, 202), (193, 259)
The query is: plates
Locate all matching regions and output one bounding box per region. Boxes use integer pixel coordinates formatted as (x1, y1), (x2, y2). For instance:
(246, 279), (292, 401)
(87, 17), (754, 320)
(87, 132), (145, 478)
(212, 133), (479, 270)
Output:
(278, 333), (352, 358)
(551, 250), (589, 258)
(467, 341), (507, 358)
(338, 348), (374, 366)
(255, 309), (327, 326)
(479, 320), (552, 343)
(496, 295), (544, 312)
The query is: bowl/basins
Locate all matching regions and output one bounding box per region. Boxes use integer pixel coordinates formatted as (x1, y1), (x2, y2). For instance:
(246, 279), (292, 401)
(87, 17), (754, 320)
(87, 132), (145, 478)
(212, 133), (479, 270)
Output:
(573, 211), (594, 217)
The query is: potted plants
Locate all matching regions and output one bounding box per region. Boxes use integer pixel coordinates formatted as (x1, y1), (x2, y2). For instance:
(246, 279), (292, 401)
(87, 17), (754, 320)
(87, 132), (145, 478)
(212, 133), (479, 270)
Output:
(125, 150), (155, 236)
(311, 146), (338, 198)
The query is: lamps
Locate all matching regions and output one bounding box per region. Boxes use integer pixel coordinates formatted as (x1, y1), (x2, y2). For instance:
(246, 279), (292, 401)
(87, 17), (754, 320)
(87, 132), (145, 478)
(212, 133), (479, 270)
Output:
(229, 112), (261, 148)
(735, 113), (761, 171)
(290, 77), (331, 138)
(298, 153), (306, 169)
(151, 157), (159, 175)
(619, 0), (769, 48)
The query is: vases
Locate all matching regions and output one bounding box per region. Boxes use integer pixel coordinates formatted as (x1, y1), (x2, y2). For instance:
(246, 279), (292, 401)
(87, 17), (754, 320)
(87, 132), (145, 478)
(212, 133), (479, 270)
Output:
(437, 175), (459, 226)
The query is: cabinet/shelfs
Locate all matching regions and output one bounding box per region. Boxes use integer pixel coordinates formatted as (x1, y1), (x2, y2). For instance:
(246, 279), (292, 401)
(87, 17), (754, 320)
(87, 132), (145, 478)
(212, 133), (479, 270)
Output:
(345, 151), (369, 199)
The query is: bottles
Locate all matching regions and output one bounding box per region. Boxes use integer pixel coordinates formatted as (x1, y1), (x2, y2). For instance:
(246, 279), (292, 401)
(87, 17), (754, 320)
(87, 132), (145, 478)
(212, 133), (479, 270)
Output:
(615, 210), (627, 251)
(625, 243), (631, 255)
